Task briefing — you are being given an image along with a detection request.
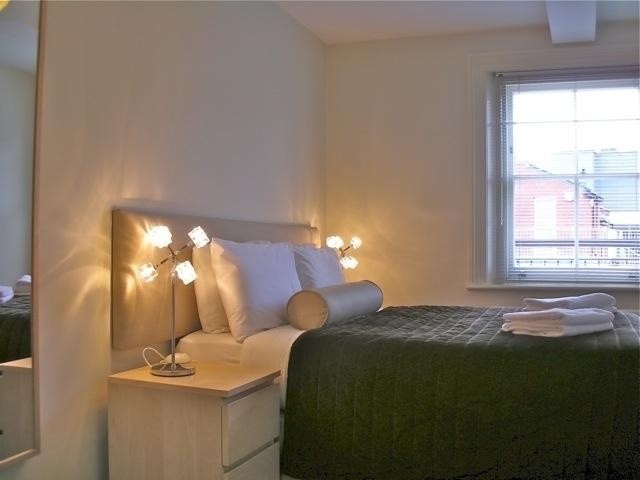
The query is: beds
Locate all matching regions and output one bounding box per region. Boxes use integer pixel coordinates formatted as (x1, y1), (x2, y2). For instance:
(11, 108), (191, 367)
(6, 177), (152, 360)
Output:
(171, 304), (640, 478)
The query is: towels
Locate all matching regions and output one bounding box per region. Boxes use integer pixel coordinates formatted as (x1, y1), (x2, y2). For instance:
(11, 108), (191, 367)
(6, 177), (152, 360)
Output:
(499, 292), (619, 338)
(0, 274), (32, 304)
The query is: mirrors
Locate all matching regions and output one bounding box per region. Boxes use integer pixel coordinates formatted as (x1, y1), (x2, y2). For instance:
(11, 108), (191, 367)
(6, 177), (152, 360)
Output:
(0, 1), (49, 471)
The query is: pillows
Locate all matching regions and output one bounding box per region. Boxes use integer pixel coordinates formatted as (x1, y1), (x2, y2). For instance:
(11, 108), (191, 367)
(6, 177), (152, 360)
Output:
(190, 234), (385, 343)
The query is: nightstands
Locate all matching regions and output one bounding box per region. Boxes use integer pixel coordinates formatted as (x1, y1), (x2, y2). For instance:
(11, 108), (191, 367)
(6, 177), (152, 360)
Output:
(105, 360), (282, 480)
